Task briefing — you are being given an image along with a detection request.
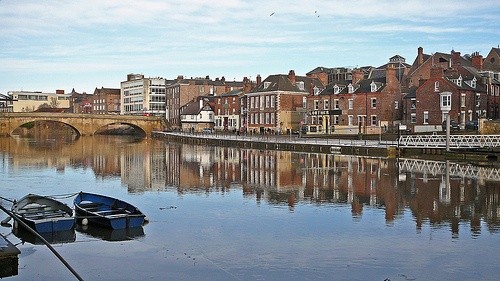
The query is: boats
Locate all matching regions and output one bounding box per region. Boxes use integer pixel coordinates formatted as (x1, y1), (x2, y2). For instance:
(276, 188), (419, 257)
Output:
(73, 190), (146, 231)
(0, 192), (75, 232)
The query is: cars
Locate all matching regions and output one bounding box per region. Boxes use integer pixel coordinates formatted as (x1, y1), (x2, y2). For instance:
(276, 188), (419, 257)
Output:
(202, 128), (215, 134)
